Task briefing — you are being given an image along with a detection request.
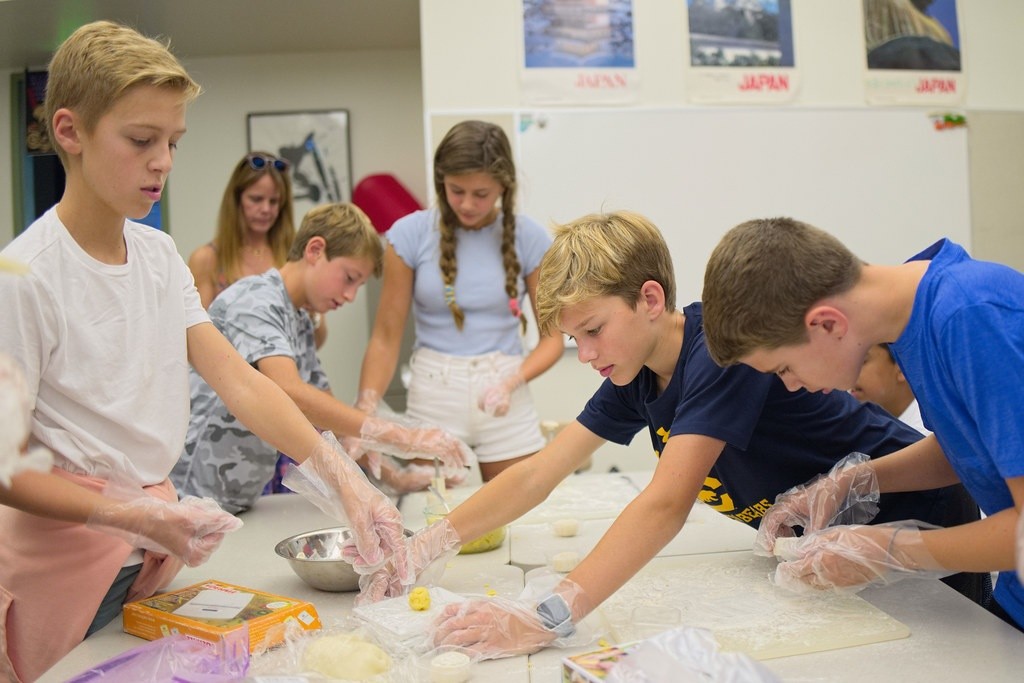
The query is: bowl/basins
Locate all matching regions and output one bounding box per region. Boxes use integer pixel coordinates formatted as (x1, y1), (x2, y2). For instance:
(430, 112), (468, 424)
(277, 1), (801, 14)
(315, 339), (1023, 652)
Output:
(417, 644), (482, 682)
(425, 502), (507, 556)
(275, 523), (416, 591)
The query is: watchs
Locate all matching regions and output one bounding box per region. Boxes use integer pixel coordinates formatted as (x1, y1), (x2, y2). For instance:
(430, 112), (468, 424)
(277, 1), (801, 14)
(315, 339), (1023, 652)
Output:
(534, 592), (577, 641)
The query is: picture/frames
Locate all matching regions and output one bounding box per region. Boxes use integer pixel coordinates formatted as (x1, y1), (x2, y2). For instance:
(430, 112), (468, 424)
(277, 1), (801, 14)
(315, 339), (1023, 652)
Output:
(246, 108), (354, 233)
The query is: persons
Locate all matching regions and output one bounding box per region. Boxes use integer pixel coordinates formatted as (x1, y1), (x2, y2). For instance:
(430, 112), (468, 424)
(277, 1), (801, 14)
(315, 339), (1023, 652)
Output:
(702, 218), (1024, 633)
(0, 21), (408, 683)
(358, 210), (994, 663)
(339, 121), (565, 492)
(188, 151), (329, 498)
(849, 343), (999, 591)
(168, 203), (467, 518)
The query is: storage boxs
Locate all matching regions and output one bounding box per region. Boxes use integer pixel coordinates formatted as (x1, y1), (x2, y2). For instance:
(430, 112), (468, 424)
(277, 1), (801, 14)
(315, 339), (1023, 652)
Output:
(121, 578), (323, 661)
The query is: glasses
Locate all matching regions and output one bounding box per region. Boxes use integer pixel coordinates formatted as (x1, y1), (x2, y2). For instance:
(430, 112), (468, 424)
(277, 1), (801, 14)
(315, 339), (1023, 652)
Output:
(244, 155), (290, 173)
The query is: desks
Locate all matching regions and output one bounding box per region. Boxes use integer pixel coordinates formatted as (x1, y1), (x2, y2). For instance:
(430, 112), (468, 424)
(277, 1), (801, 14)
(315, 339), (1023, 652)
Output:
(156, 486), (1024, 683)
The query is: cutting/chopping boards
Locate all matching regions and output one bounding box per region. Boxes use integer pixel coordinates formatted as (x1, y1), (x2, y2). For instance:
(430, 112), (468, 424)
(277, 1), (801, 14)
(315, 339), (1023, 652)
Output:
(595, 551), (912, 661)
